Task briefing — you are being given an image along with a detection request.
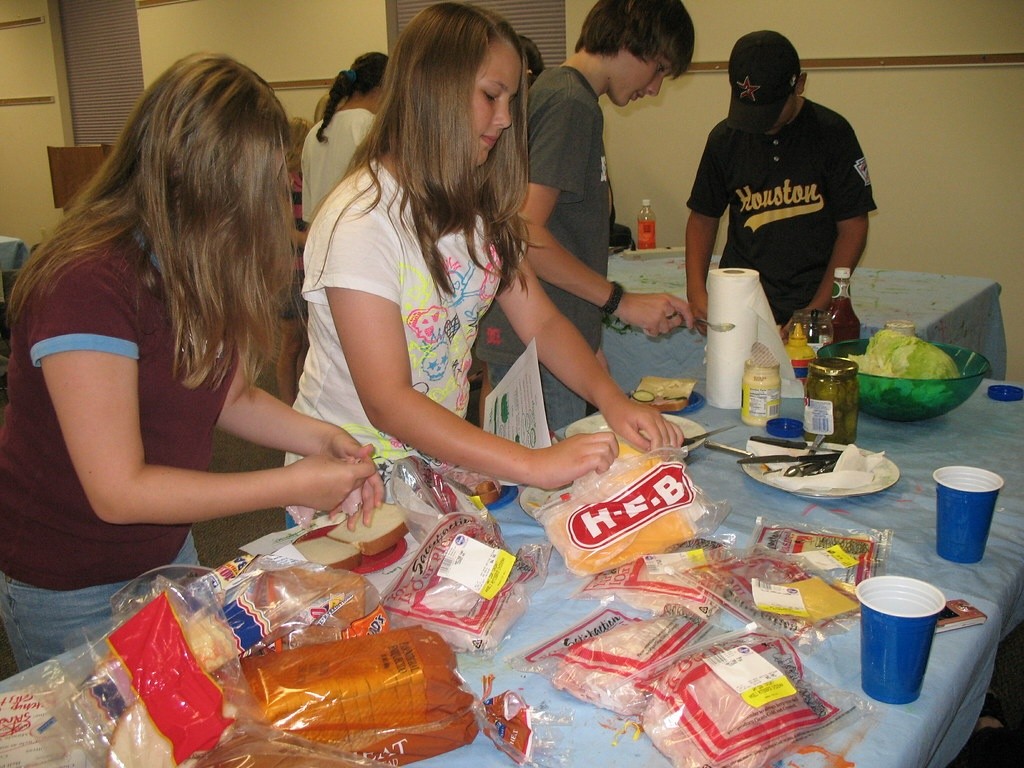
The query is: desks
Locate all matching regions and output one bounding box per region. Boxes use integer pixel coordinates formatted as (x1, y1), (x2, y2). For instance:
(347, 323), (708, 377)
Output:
(0, 376), (1023, 768)
(601, 251), (1007, 400)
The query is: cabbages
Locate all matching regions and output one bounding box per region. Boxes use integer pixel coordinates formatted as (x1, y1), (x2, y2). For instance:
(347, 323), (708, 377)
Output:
(849, 330), (959, 411)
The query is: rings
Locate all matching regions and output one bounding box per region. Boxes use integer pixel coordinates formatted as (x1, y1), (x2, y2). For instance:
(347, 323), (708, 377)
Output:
(665, 310), (678, 319)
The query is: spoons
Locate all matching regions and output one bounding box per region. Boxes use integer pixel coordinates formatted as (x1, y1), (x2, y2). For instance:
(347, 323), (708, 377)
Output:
(678, 310), (735, 332)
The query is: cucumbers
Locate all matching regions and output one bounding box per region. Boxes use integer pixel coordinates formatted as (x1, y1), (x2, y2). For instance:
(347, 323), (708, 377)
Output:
(632, 390), (654, 402)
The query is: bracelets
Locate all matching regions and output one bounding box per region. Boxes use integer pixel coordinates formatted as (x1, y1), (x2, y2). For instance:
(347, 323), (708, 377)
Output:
(601, 281), (623, 314)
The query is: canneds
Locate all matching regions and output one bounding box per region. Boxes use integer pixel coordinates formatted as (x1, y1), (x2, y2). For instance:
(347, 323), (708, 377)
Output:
(803, 358), (858, 446)
(789, 307), (833, 355)
(741, 361), (782, 428)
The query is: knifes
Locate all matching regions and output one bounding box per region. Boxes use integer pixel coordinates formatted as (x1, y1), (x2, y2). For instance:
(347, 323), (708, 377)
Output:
(751, 435), (844, 453)
(681, 424), (737, 448)
(736, 453), (842, 464)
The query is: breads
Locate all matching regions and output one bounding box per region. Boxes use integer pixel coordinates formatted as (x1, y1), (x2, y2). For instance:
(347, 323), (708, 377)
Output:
(108, 571), (480, 768)
(630, 396), (688, 411)
(293, 500), (408, 570)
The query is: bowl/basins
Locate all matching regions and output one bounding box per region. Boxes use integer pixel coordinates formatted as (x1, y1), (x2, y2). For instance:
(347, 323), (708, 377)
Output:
(817, 338), (990, 422)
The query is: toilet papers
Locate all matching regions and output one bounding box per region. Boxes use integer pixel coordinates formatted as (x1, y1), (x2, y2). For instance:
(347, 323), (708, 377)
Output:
(705, 267), (806, 411)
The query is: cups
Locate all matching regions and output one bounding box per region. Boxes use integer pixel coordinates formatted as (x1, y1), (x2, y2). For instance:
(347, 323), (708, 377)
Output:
(855, 575), (946, 704)
(932, 465), (1005, 563)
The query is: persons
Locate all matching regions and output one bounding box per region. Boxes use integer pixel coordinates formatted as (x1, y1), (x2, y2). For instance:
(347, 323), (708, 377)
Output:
(282, 4), (686, 555)
(471, 0), (695, 449)
(0, 51), (386, 687)
(272, 34), (546, 431)
(685, 30), (877, 344)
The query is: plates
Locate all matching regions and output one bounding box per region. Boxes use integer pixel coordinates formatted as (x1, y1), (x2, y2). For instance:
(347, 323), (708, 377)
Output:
(627, 391), (705, 415)
(292, 523), (407, 574)
(565, 411), (705, 452)
(742, 441), (900, 499)
(484, 485), (519, 509)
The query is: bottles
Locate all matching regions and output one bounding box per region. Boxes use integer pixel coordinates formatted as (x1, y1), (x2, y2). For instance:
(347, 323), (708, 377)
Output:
(638, 199), (656, 249)
(828, 267), (860, 343)
(741, 341), (781, 427)
(783, 309), (833, 408)
(804, 358), (858, 444)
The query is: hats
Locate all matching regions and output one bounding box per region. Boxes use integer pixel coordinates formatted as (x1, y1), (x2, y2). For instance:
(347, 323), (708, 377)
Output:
(725, 31), (800, 133)
